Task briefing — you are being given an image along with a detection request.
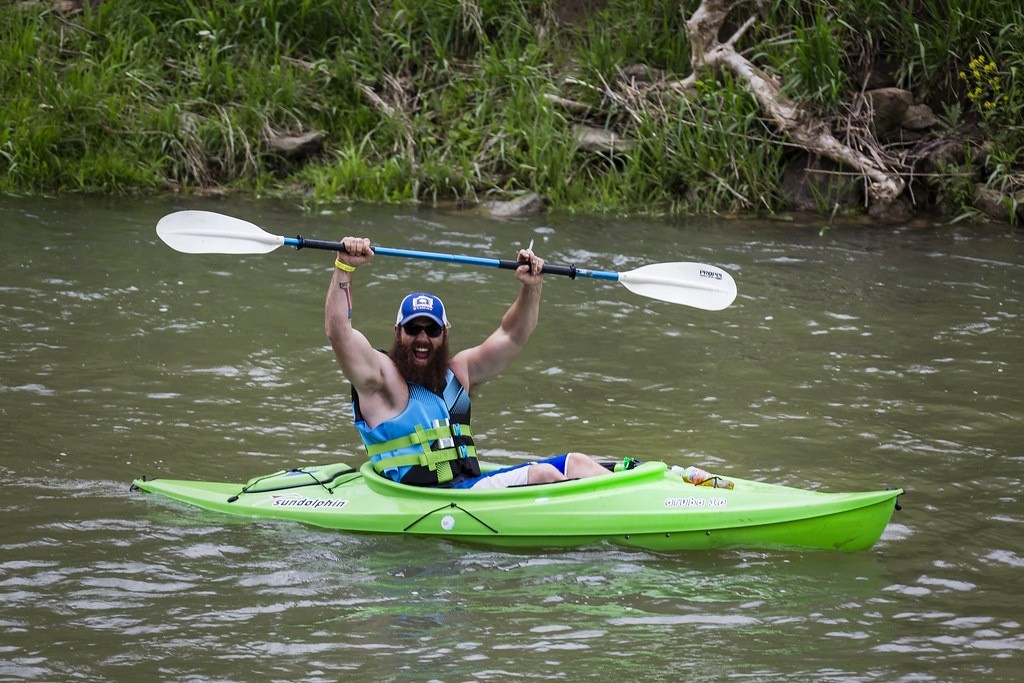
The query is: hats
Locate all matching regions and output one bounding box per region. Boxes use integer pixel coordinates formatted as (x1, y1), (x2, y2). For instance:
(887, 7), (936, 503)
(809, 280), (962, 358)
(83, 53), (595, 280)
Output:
(396, 293), (448, 327)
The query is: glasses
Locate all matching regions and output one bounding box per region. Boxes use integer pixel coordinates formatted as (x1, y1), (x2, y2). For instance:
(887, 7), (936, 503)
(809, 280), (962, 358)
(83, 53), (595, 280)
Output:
(402, 322), (443, 338)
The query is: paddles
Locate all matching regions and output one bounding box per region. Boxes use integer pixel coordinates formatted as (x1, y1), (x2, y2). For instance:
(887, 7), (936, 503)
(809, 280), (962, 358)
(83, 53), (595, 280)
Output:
(156, 210), (737, 311)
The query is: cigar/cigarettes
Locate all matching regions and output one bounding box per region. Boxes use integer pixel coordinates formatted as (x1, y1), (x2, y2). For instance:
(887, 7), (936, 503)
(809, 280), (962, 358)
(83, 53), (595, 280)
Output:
(525, 239), (535, 260)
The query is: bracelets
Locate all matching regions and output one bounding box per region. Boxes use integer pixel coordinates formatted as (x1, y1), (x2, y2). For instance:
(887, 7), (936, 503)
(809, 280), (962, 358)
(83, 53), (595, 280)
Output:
(333, 258), (356, 273)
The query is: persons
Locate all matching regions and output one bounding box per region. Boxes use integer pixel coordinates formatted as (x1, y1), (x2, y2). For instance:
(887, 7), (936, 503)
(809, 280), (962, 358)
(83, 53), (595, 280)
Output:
(326, 237), (614, 492)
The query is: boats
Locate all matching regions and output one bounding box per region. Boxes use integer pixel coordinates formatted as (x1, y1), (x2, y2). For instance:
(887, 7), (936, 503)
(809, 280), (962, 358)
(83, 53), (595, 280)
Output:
(131, 462), (905, 550)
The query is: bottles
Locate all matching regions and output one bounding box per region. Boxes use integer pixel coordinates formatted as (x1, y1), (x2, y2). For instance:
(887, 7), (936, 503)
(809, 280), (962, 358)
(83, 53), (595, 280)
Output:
(599, 457), (635, 474)
(671, 465), (735, 490)
